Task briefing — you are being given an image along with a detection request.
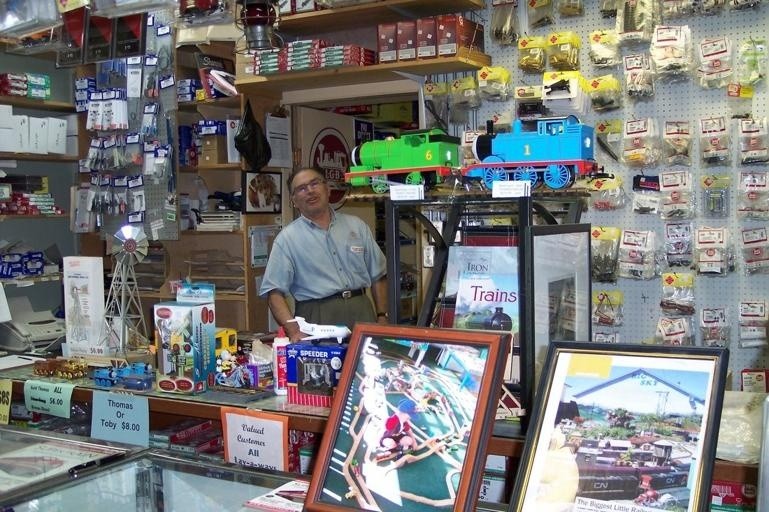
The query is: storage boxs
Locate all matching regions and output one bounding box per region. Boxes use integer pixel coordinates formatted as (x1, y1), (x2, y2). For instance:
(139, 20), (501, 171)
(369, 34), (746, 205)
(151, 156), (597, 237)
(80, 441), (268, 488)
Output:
(707, 459), (759, 512)
(150, 280), (349, 408)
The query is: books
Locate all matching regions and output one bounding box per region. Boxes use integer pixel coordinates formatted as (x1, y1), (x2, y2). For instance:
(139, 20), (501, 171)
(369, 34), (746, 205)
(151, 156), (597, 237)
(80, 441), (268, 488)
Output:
(196, 212), (239, 232)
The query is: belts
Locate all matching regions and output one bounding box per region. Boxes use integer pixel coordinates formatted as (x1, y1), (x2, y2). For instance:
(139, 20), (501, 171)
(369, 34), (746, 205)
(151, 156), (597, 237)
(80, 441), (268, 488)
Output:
(327, 289), (366, 299)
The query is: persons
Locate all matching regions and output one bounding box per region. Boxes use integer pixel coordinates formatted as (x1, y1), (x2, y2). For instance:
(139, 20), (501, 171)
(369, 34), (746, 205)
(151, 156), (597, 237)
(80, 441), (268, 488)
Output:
(258, 167), (390, 343)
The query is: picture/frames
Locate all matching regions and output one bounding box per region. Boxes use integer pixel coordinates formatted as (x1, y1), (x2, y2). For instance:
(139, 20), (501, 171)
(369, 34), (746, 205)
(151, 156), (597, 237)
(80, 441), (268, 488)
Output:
(507, 337), (729, 511)
(299, 319), (517, 512)
(518, 220), (592, 437)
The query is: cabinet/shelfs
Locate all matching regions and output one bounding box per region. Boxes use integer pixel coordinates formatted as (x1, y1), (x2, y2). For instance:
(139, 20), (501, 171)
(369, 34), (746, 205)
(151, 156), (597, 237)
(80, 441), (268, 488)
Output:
(230, 1), (496, 92)
(72, 34), (279, 336)
(0, 44), (79, 366)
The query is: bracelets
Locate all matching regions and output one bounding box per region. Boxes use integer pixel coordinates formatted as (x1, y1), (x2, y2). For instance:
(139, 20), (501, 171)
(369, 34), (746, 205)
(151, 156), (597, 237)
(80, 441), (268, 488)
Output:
(376, 312), (389, 318)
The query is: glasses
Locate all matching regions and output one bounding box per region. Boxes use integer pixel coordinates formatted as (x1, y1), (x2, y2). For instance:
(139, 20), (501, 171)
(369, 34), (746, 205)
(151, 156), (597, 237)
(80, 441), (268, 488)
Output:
(290, 178), (326, 201)
